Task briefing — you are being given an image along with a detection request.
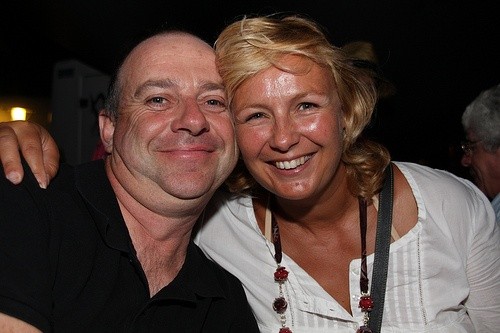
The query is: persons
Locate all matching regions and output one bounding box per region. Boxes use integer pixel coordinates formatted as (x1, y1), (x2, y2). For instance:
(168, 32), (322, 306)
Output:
(1, 12), (499, 333)
(0, 28), (260, 333)
(459, 84), (500, 229)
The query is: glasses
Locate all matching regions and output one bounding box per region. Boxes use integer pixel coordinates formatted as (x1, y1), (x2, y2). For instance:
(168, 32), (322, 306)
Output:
(458, 136), (485, 158)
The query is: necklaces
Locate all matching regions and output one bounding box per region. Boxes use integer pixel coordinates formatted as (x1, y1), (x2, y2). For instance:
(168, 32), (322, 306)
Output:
(271, 195), (373, 333)
